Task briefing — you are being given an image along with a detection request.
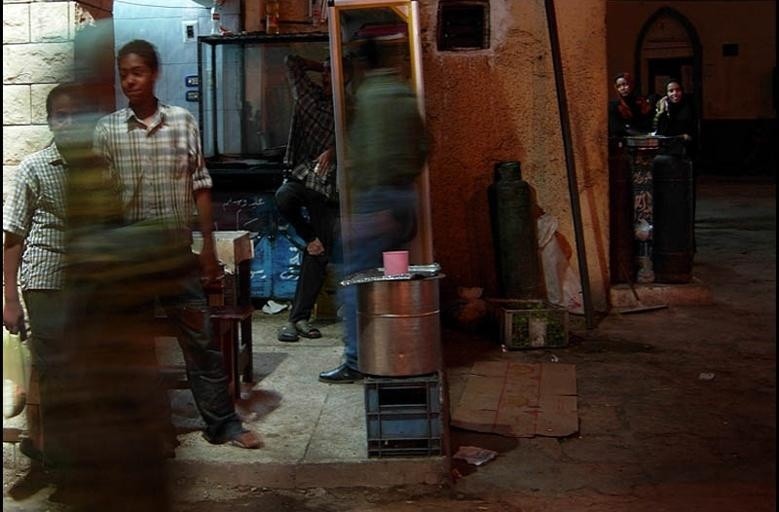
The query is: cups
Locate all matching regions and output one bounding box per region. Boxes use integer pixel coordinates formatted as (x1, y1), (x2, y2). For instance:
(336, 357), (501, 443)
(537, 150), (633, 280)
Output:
(381, 251), (409, 277)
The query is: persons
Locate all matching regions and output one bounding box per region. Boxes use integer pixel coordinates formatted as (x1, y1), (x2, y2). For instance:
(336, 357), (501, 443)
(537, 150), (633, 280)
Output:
(609, 70), (654, 135)
(2, 81), (127, 506)
(652, 81), (704, 179)
(316, 39), (430, 386)
(94, 43), (263, 452)
(271, 51), (355, 340)
(33, 159), (201, 511)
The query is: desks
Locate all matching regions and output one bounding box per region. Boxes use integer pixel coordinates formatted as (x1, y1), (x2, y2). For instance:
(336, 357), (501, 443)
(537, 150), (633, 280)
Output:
(186, 227), (255, 399)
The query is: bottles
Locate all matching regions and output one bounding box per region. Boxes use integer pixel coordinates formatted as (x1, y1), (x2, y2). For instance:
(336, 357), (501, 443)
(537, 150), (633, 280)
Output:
(266, 0), (281, 35)
(209, 0), (221, 35)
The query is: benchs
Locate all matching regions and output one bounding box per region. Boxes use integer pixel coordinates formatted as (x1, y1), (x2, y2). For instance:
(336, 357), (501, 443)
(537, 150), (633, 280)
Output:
(148, 307), (254, 399)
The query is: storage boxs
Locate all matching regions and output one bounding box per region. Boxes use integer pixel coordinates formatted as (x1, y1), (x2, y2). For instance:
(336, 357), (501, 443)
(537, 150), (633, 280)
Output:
(361, 374), (447, 457)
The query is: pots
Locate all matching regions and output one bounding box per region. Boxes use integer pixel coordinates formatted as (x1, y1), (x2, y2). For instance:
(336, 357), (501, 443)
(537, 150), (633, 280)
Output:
(263, 143), (288, 163)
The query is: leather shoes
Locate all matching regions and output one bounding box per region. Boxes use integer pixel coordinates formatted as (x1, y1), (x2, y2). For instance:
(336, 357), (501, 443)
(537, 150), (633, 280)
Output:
(230, 431), (261, 449)
(278, 321), (298, 341)
(319, 362), (362, 383)
(297, 320), (322, 338)
